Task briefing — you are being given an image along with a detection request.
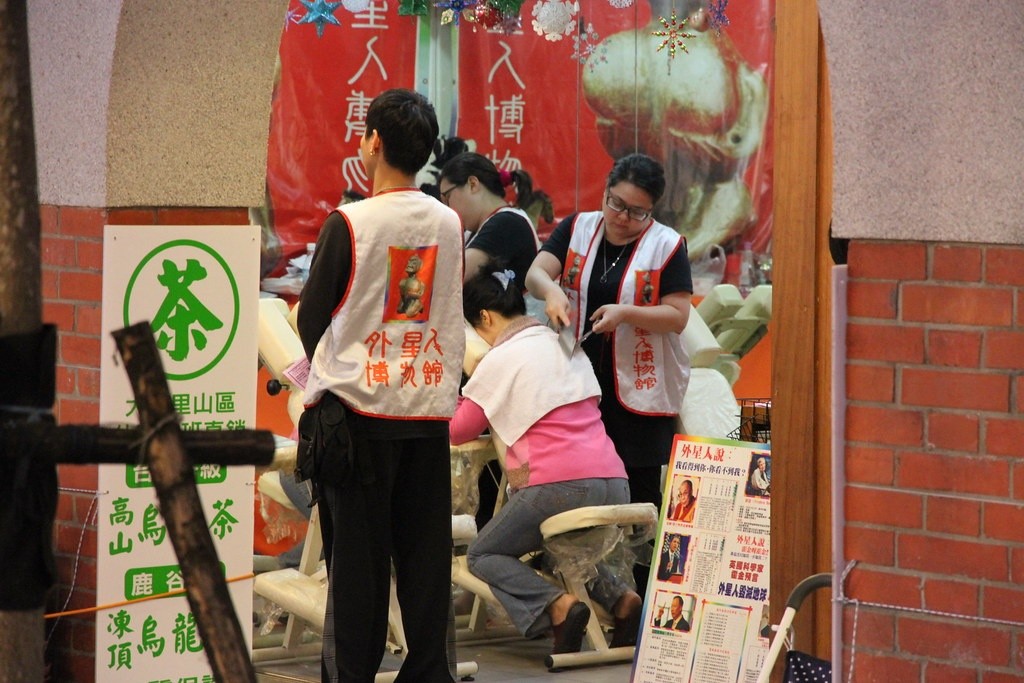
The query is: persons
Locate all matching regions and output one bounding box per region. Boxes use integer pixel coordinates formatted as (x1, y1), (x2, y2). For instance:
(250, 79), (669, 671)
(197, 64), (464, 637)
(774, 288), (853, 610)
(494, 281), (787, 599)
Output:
(448, 267), (643, 653)
(761, 613), (770, 638)
(441, 152), (553, 627)
(295, 88), (466, 683)
(752, 458), (769, 496)
(671, 480), (697, 523)
(526, 154), (693, 603)
(654, 596), (689, 631)
(658, 537), (681, 581)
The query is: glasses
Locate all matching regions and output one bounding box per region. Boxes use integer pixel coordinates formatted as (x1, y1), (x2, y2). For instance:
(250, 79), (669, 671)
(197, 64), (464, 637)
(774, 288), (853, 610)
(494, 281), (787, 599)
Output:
(439, 185), (458, 206)
(606, 185), (653, 222)
(678, 492), (688, 498)
(671, 554), (674, 562)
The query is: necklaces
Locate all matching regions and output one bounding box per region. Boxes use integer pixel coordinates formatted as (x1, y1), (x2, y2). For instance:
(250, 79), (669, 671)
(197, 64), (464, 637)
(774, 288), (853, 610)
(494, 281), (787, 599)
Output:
(600, 222), (630, 284)
(372, 186), (419, 197)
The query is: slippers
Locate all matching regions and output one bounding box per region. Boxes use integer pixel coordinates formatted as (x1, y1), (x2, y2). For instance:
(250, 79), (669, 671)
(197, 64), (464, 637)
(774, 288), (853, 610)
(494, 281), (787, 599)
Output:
(550, 602), (591, 654)
(608, 605), (643, 649)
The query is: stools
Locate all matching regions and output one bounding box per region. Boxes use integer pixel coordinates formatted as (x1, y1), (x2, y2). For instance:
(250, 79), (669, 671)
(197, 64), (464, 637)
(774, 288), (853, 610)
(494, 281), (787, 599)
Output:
(538, 502), (660, 670)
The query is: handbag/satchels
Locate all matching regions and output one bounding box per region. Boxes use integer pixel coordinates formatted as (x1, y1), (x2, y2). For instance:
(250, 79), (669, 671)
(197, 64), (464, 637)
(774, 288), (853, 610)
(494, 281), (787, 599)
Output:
(293, 401), (352, 483)
